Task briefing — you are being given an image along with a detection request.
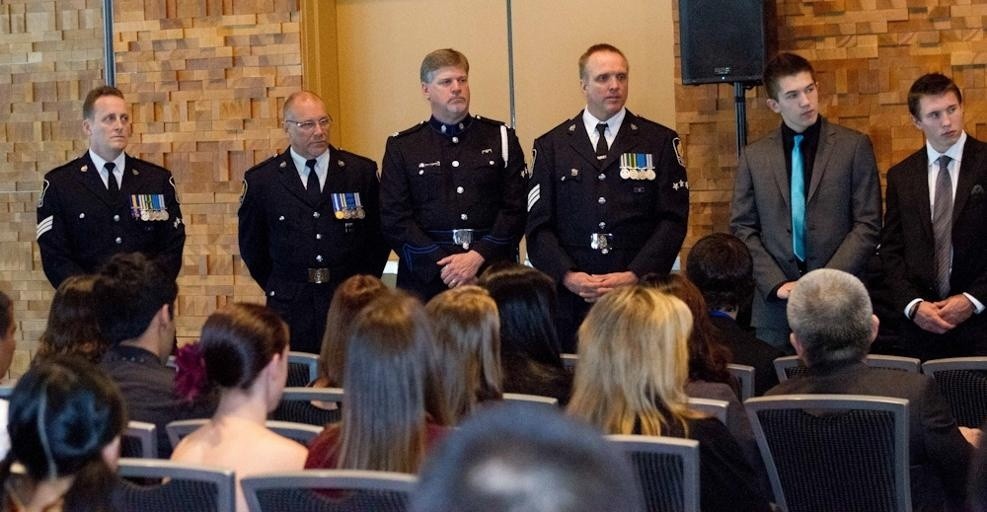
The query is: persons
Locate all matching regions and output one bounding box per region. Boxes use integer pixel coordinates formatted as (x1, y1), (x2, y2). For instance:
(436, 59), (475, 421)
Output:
(726, 52), (884, 353)
(237, 91), (392, 355)
(879, 72), (987, 361)
(35, 86), (185, 354)
(525, 44), (689, 317)
(0, 233), (987, 512)
(377, 48), (527, 302)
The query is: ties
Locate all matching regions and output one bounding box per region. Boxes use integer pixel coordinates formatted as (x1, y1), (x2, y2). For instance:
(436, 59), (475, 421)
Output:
(932, 155), (955, 298)
(595, 123), (608, 164)
(790, 136), (808, 262)
(105, 161), (120, 193)
(305, 159), (321, 198)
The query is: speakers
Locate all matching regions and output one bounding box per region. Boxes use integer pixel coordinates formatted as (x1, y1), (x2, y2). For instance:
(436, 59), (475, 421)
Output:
(679, 0), (768, 86)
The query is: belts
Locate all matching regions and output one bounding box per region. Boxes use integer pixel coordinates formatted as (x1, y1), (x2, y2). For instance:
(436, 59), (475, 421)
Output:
(301, 267), (331, 284)
(566, 230), (634, 250)
(430, 229), (485, 245)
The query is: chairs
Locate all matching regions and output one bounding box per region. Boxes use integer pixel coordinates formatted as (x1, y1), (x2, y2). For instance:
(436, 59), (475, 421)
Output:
(0, 333), (985, 510)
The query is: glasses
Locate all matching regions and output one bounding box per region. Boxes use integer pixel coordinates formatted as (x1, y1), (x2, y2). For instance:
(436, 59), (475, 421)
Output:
(287, 113), (331, 129)
